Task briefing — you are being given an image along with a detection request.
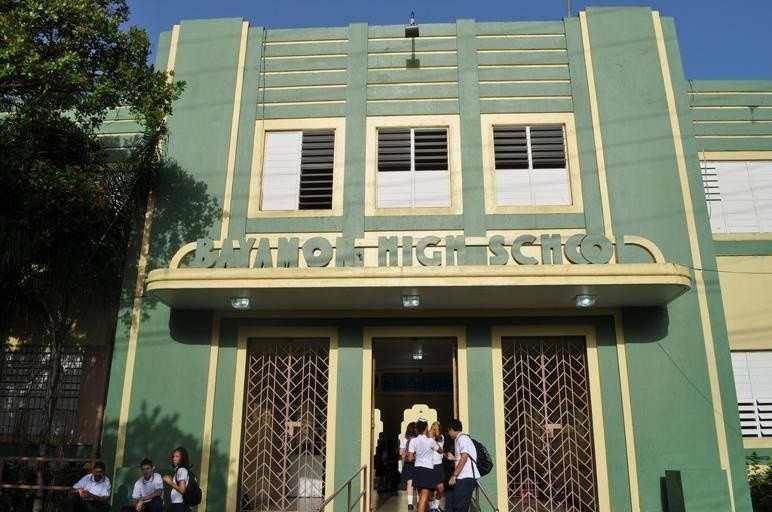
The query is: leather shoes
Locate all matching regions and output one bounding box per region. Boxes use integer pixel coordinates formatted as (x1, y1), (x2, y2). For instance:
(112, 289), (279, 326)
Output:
(408, 505), (414, 510)
(429, 508), (442, 512)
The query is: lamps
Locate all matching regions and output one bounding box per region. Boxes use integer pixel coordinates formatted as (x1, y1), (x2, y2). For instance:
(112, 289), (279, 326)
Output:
(228, 296), (252, 310)
(400, 294), (421, 310)
(404, 12), (419, 38)
(572, 293), (597, 307)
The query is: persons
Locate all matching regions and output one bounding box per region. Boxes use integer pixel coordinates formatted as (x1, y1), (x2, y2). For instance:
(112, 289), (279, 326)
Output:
(120, 458), (165, 512)
(374, 419), (482, 512)
(161, 446), (198, 511)
(68, 461), (111, 512)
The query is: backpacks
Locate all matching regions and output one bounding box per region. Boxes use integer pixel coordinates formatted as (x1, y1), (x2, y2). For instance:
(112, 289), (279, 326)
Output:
(174, 466), (202, 505)
(456, 434), (494, 480)
